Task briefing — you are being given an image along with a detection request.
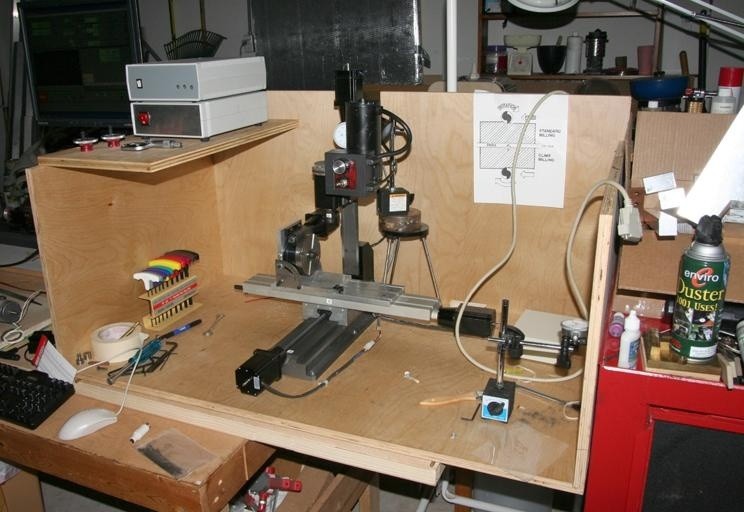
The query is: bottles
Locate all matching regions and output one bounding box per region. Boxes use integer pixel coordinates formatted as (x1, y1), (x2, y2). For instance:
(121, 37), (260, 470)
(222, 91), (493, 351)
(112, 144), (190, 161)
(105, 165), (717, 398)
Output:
(618, 310), (641, 368)
(717, 66), (744, 107)
(709, 88), (736, 115)
(484, 45), (508, 75)
(565, 32), (582, 75)
(609, 312), (625, 338)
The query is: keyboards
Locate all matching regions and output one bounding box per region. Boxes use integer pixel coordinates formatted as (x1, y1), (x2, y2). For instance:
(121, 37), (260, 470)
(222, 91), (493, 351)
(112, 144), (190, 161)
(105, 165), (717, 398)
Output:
(0, 362), (75, 430)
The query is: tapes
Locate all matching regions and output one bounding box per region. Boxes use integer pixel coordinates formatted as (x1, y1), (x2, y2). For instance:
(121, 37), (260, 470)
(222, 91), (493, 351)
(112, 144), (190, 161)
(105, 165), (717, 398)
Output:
(90, 321), (141, 364)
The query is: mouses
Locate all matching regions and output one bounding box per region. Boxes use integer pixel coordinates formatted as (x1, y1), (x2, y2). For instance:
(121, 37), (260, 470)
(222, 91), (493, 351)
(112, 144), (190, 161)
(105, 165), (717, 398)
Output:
(58, 406), (118, 442)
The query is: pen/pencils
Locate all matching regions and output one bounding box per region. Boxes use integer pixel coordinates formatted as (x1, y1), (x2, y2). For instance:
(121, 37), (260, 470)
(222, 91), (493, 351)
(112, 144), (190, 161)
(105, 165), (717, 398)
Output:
(158, 318), (203, 341)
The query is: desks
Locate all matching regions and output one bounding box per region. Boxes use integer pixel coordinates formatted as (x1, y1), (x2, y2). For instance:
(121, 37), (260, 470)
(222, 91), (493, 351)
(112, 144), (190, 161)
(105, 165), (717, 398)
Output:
(0, 90), (631, 511)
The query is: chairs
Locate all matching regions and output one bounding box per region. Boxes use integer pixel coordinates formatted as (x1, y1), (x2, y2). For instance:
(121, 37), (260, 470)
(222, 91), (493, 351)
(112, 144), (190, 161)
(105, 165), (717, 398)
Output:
(13, 1), (160, 163)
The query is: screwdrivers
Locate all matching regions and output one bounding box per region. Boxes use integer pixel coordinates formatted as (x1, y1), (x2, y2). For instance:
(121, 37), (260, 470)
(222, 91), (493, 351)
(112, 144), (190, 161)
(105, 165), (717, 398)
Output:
(107, 340), (161, 385)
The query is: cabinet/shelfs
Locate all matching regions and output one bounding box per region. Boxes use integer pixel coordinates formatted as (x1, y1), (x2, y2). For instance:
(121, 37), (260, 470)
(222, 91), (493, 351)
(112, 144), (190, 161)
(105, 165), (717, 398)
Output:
(581, 112), (743, 512)
(477, 1), (664, 92)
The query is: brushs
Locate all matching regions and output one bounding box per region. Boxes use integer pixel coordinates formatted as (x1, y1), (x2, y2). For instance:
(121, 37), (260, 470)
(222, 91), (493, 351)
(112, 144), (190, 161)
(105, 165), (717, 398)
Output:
(419, 388), (484, 406)
